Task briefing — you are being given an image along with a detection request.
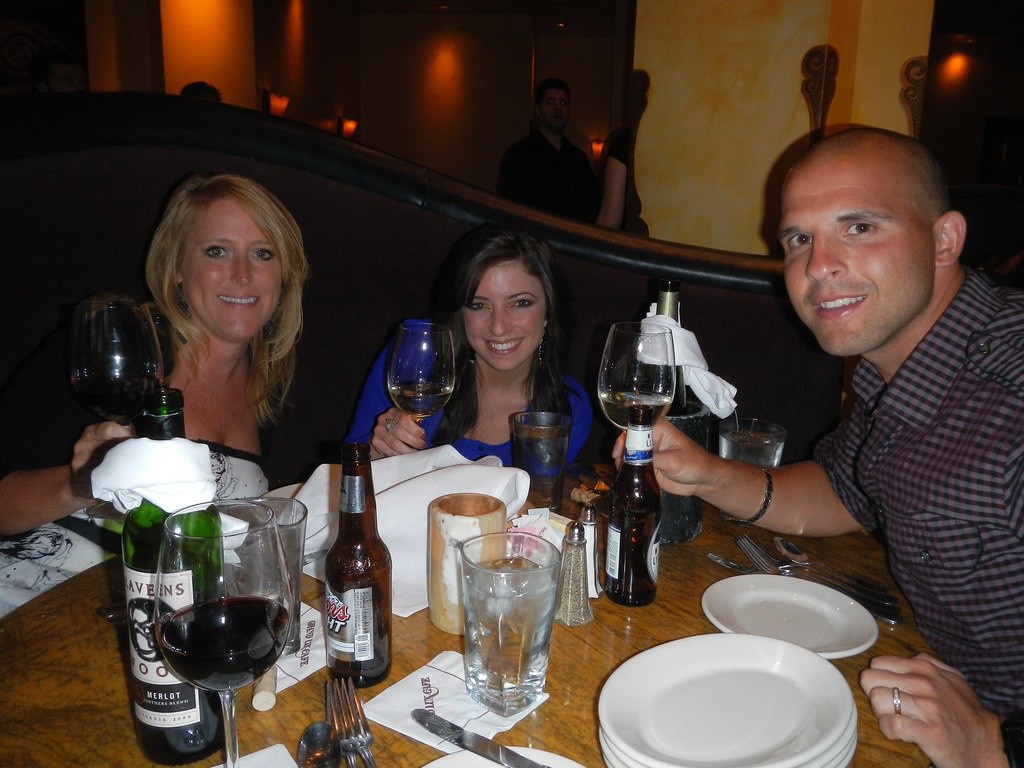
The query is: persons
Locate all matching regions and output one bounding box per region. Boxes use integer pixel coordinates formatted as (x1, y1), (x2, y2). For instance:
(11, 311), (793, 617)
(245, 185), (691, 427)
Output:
(181, 83), (219, 102)
(340, 217), (593, 471)
(29, 43), (90, 93)
(495, 77), (630, 232)
(0, 170), (341, 537)
(610, 124), (1024, 768)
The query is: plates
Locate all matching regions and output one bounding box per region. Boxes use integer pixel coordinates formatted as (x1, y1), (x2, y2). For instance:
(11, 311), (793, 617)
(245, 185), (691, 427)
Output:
(421, 745), (587, 768)
(598, 632), (858, 768)
(701, 572), (878, 659)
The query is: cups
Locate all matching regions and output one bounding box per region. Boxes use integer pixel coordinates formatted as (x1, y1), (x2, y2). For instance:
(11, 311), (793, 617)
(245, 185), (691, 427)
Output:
(512, 410), (571, 512)
(429, 492), (507, 635)
(75, 299), (164, 426)
(240, 497), (308, 657)
(720, 418), (786, 524)
(457, 530), (562, 717)
(387, 322), (456, 426)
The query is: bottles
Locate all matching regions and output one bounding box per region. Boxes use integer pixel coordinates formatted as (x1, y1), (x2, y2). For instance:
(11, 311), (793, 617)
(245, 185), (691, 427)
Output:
(324, 442), (392, 687)
(552, 521), (593, 627)
(604, 405), (663, 606)
(657, 280), (687, 416)
(120, 386), (222, 764)
(577, 504), (603, 599)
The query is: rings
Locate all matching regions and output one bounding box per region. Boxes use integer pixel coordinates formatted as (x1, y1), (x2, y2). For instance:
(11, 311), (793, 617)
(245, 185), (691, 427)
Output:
(385, 417), (397, 430)
(893, 688), (904, 715)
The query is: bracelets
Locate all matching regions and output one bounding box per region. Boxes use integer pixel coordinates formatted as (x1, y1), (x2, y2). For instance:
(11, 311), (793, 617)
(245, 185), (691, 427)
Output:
(728, 468), (772, 526)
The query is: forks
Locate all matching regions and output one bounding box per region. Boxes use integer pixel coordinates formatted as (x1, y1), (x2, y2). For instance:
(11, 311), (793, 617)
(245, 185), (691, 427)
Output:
(735, 532), (903, 626)
(324, 677), (377, 768)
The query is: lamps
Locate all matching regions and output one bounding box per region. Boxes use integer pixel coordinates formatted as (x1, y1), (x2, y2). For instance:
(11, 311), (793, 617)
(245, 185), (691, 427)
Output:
(590, 138), (605, 162)
(262, 88), (289, 118)
(942, 33), (978, 55)
(337, 115), (358, 141)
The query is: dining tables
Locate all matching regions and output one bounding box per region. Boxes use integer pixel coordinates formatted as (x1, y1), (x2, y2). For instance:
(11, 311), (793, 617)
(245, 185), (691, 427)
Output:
(0, 462), (947, 768)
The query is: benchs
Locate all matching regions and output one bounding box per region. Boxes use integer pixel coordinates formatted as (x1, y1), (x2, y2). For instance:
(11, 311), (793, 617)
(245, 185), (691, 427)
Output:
(0, 92), (865, 489)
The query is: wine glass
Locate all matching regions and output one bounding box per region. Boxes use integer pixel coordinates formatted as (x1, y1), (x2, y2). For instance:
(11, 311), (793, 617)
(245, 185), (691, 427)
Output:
(593, 320), (676, 519)
(153, 499), (296, 768)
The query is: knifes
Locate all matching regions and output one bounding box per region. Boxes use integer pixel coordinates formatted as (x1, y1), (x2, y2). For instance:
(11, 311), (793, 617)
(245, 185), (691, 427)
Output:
(411, 708), (552, 768)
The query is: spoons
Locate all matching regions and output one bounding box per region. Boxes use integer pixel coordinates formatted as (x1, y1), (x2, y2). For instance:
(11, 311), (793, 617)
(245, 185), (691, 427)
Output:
(772, 535), (890, 595)
(296, 721), (341, 768)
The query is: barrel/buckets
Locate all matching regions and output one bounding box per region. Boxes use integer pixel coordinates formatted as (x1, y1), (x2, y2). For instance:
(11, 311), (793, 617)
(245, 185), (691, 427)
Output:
(655, 400), (711, 543)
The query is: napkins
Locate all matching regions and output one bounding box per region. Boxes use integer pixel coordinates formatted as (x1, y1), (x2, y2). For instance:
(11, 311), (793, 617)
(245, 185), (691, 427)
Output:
(360, 650), (550, 755)
